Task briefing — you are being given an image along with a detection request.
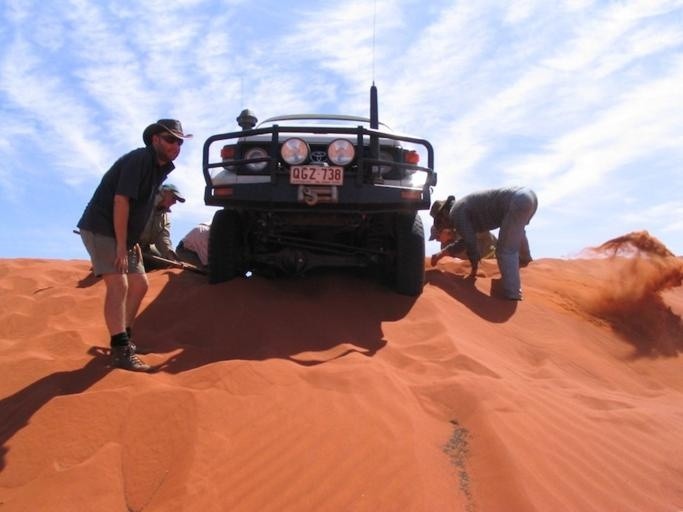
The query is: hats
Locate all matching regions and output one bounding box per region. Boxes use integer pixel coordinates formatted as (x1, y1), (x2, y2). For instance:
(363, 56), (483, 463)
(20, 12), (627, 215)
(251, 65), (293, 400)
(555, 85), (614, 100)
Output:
(430, 195), (455, 227)
(143, 119), (193, 145)
(163, 184), (187, 204)
(429, 225), (443, 240)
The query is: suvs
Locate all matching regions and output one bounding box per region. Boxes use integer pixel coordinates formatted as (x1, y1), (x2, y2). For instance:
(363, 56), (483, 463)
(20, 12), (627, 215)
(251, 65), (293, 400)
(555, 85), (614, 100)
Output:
(202, 80), (438, 296)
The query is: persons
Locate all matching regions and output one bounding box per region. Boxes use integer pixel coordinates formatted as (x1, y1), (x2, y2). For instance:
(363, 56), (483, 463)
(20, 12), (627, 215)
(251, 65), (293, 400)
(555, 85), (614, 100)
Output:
(430, 187), (538, 301)
(429, 225), (497, 260)
(77, 119), (193, 373)
(137, 184), (190, 272)
(175, 223), (211, 268)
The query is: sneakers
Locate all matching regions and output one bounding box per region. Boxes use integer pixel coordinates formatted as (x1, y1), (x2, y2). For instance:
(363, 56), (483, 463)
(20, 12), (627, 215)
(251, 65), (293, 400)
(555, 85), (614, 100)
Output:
(113, 342), (156, 373)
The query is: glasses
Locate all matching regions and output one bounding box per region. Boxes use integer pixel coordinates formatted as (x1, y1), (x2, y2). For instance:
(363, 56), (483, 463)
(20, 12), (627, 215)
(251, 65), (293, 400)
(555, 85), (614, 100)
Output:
(160, 133), (183, 146)
